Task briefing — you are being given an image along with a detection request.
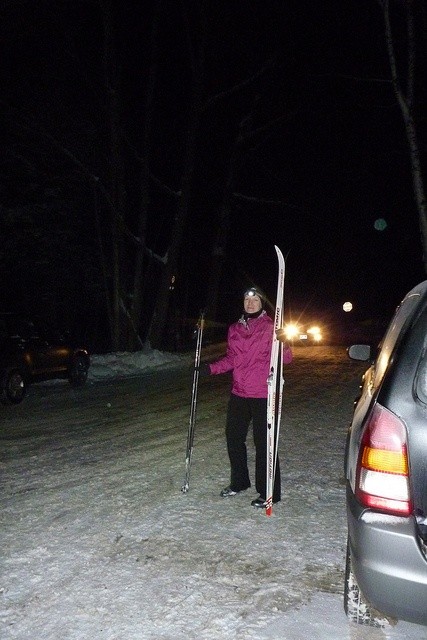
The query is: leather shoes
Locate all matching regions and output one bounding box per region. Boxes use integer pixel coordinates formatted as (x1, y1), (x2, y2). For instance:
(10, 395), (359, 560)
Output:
(275, 329), (286, 342)
(192, 364), (211, 378)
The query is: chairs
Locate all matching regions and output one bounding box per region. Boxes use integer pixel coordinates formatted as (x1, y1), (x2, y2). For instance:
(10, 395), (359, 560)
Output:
(220, 485), (240, 497)
(251, 494), (266, 508)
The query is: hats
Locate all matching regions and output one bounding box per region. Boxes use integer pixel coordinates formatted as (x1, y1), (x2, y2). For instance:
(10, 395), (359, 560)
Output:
(280, 310), (329, 345)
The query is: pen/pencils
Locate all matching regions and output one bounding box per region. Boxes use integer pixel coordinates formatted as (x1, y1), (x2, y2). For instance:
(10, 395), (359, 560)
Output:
(346, 279), (427, 629)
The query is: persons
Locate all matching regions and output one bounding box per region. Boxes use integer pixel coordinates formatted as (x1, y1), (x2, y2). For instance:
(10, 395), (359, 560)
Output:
(188, 282), (294, 509)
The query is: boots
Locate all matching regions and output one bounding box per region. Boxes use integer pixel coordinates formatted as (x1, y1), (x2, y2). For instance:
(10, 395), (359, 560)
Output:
(3, 309), (91, 406)
